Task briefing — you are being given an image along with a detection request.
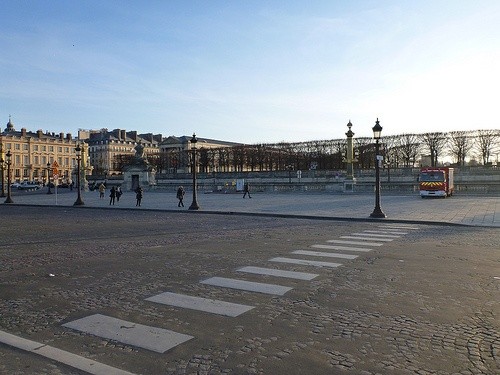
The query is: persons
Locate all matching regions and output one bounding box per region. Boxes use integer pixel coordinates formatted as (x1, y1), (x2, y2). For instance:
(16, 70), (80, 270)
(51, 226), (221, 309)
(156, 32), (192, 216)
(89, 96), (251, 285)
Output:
(109, 187), (116, 205)
(135, 185), (143, 207)
(99, 182), (106, 199)
(176, 186), (185, 207)
(336, 171), (339, 178)
(116, 185), (123, 202)
(243, 182), (253, 198)
(70, 183), (73, 190)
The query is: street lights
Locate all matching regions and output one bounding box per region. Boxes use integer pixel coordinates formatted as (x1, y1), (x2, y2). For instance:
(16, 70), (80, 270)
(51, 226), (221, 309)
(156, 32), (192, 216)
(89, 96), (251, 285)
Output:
(210, 164), (219, 192)
(285, 164), (294, 188)
(4, 150), (15, 203)
(369, 117), (388, 218)
(104, 170), (107, 187)
(188, 132), (201, 210)
(73, 143), (86, 205)
(384, 160), (395, 191)
(0, 158), (7, 198)
(46, 161), (53, 194)
(344, 120), (356, 180)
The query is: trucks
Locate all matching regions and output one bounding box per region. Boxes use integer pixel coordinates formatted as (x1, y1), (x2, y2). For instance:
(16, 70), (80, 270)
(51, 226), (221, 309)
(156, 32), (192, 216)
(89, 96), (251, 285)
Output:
(417, 167), (455, 199)
